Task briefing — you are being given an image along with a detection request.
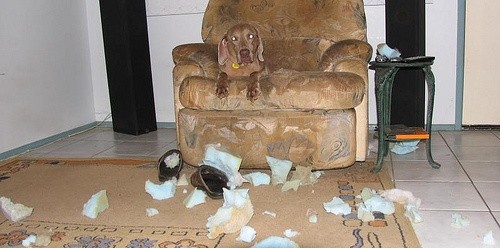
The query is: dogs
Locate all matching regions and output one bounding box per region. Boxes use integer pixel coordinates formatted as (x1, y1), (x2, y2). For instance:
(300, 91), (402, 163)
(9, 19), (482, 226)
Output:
(214, 23), (270, 103)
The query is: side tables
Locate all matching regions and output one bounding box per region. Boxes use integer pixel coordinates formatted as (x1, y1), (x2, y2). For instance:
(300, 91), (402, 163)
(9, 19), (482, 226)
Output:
(368, 55), (441, 172)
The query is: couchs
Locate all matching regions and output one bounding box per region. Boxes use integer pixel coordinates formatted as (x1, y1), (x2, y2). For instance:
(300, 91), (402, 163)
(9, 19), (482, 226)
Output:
(170, 1), (373, 172)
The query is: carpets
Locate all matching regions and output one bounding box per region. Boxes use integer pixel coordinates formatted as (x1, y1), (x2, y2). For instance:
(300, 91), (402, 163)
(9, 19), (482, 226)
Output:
(0, 157), (422, 248)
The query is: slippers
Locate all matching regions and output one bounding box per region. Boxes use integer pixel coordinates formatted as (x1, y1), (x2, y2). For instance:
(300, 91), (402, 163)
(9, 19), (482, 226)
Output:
(156, 149), (184, 180)
(192, 166), (230, 198)
